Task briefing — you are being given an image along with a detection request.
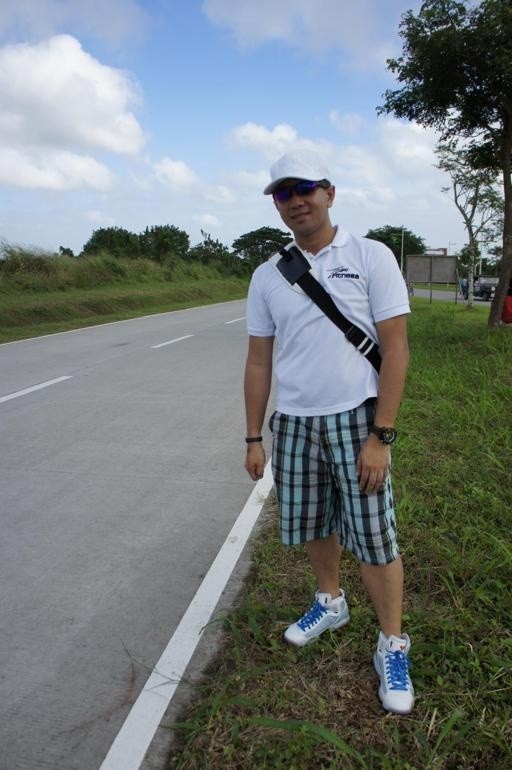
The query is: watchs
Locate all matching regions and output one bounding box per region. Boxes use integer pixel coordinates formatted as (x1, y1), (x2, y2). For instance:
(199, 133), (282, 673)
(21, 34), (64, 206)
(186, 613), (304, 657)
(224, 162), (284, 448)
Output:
(372, 424), (397, 445)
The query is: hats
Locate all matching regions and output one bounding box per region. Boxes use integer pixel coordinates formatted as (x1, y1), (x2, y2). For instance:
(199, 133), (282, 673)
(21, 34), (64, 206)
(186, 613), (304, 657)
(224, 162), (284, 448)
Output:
(263, 147), (333, 195)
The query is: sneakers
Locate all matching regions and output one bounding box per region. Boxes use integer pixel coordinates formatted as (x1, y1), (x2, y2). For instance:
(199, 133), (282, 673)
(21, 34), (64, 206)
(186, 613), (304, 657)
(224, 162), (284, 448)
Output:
(284, 588), (350, 646)
(373, 631), (414, 714)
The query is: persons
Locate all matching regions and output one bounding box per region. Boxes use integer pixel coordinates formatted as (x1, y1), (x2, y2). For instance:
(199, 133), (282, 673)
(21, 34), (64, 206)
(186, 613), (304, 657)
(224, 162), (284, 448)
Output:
(242, 146), (413, 714)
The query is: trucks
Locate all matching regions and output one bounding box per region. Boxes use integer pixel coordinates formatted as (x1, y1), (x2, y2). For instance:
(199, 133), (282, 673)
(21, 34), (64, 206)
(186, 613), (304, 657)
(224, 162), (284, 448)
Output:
(461, 274), (500, 301)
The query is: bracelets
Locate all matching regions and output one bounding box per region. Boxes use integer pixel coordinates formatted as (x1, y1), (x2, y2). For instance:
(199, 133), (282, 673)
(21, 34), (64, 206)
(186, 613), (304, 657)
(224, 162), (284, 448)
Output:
(246, 436), (262, 443)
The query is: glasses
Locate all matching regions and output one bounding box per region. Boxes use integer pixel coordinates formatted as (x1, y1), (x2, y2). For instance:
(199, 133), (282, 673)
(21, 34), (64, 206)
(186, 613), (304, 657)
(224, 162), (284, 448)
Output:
(273, 181), (325, 202)
